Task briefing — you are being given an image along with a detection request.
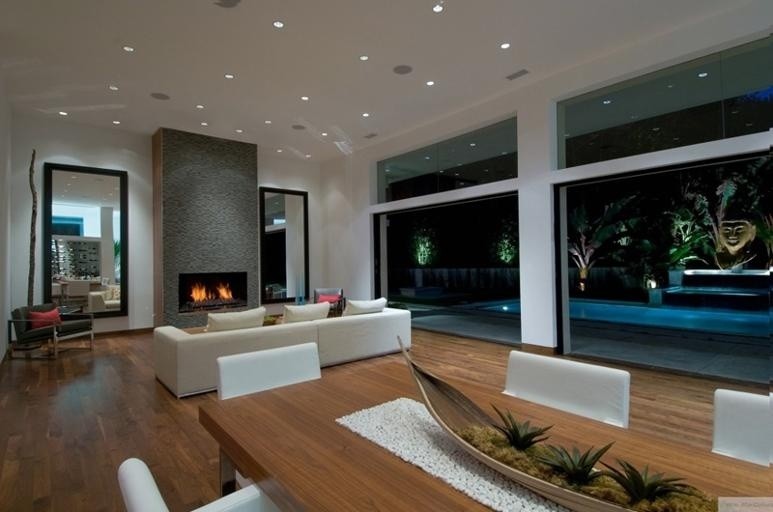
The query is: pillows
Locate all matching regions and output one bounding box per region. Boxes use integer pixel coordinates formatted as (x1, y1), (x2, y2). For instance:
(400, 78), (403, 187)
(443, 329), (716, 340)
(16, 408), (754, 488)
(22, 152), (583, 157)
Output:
(30, 308), (62, 328)
(106, 288), (120, 300)
(317, 295), (340, 304)
(204, 297), (388, 332)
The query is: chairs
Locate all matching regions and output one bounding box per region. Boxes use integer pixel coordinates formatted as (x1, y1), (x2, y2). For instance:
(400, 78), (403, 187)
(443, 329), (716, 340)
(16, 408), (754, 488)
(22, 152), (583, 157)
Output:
(51, 277), (111, 308)
(216, 342), (322, 399)
(118, 458), (279, 512)
(501, 351), (630, 428)
(7, 302), (94, 360)
(314, 288), (346, 317)
(711, 387), (771, 464)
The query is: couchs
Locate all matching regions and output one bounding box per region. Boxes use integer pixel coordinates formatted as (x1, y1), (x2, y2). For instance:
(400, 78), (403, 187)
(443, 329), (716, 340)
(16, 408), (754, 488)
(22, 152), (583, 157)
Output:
(154, 307), (411, 400)
(88, 289), (121, 312)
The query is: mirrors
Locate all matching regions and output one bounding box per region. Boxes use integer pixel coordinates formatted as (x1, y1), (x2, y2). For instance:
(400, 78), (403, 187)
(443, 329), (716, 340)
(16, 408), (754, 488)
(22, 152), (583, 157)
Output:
(259, 186), (311, 305)
(42, 162), (129, 322)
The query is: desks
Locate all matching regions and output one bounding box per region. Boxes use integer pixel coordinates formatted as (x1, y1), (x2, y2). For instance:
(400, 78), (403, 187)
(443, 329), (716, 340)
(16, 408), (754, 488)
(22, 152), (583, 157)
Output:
(199, 356), (771, 510)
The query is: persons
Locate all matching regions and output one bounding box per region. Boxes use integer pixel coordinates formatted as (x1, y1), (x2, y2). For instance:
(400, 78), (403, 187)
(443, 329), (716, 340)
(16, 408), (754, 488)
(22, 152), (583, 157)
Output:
(714, 208), (759, 271)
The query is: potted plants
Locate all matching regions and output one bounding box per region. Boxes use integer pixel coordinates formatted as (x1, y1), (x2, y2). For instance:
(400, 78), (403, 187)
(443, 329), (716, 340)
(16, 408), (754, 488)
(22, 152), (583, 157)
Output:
(400, 344), (717, 511)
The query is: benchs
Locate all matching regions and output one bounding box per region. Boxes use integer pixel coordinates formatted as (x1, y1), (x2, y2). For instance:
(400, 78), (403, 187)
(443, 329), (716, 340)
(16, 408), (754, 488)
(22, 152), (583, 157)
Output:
(647, 286), (771, 311)
